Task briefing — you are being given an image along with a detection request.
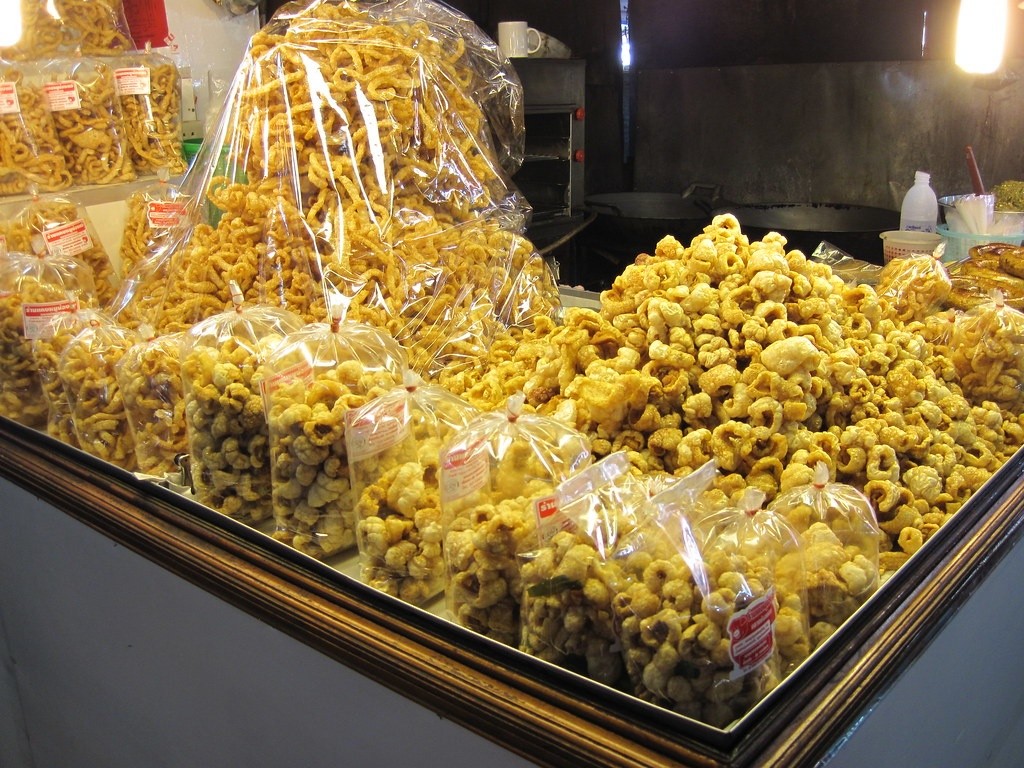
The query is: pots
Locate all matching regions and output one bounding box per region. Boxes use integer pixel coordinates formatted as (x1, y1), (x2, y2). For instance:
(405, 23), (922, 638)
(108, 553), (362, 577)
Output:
(584, 183), (725, 237)
(693, 197), (901, 262)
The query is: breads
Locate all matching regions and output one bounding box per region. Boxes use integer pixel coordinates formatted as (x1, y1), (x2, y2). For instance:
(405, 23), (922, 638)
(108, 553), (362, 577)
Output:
(942, 242), (1024, 312)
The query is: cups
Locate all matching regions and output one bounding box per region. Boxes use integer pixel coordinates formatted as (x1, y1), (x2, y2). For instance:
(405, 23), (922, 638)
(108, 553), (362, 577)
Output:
(498, 22), (541, 59)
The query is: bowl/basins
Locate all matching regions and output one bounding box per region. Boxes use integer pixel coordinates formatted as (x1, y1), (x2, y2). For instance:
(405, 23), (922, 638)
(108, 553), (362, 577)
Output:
(938, 195), (1024, 224)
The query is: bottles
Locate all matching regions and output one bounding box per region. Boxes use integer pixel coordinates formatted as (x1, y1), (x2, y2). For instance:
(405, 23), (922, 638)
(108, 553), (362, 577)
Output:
(900, 170), (937, 234)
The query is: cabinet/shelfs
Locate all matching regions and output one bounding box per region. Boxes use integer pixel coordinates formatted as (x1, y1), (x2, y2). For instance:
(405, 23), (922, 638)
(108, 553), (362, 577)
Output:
(507, 58), (585, 241)
